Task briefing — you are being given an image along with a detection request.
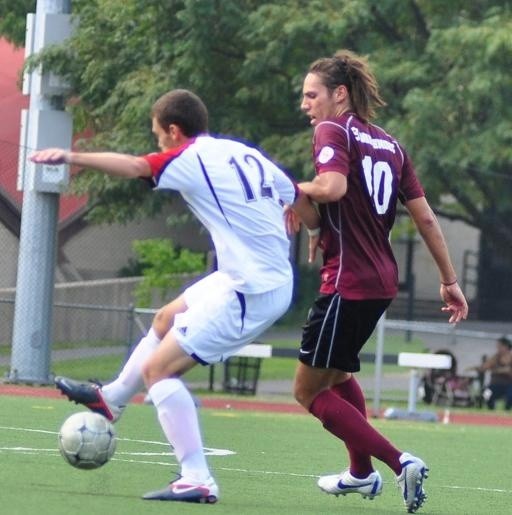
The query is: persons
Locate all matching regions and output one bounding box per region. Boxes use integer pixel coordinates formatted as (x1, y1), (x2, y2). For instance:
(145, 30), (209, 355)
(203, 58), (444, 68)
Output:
(466, 339), (512, 410)
(285, 50), (469, 514)
(445, 376), (468, 389)
(29, 88), (321, 505)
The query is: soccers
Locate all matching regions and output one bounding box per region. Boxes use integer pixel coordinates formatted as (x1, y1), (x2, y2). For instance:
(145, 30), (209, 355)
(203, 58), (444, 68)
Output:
(59, 412), (116, 469)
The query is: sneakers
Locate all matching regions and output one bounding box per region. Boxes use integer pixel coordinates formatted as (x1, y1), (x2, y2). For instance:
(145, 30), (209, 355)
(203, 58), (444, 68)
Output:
(317, 466), (384, 500)
(141, 470), (220, 504)
(53, 375), (126, 425)
(396, 452), (430, 514)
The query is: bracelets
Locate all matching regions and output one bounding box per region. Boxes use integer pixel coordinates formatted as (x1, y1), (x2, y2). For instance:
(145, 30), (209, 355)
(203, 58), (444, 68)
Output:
(439, 279), (458, 287)
(306, 227), (321, 237)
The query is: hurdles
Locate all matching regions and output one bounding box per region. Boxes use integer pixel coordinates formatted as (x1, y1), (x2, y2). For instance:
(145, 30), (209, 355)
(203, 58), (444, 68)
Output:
(232, 343), (452, 422)
